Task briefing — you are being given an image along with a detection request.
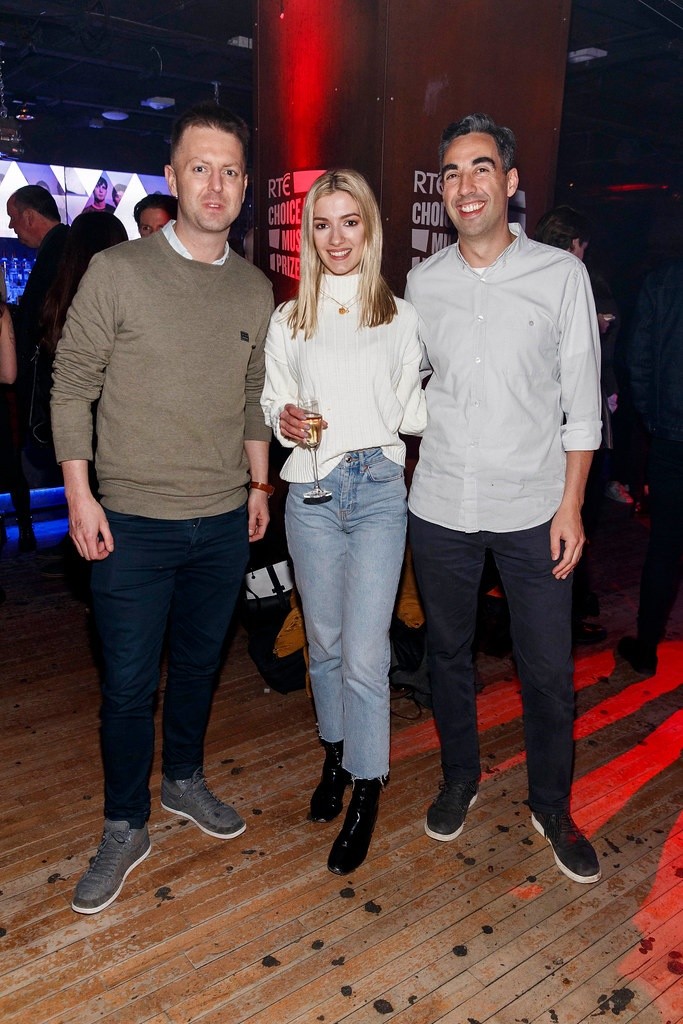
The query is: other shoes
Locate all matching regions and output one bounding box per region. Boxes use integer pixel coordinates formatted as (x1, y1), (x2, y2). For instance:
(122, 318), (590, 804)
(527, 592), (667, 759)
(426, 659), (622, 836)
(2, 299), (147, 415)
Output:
(619, 637), (657, 677)
(39, 558), (75, 577)
(35, 541), (66, 560)
(574, 622), (607, 644)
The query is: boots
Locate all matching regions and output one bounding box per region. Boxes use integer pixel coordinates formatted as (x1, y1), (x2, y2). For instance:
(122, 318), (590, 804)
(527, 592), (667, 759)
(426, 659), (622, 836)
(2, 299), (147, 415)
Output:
(310, 742), (350, 823)
(16, 515), (37, 555)
(327, 779), (382, 874)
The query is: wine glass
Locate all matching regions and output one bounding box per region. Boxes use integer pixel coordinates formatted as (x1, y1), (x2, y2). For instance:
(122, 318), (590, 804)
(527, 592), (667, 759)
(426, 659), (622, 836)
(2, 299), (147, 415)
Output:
(300, 400), (333, 498)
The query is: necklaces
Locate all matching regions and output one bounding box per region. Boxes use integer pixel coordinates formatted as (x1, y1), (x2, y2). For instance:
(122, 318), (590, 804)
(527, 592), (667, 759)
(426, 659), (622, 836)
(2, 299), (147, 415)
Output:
(319, 288), (360, 314)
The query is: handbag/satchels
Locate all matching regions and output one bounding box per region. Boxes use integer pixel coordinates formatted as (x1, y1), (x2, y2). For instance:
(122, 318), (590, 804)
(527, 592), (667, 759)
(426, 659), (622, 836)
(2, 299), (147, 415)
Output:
(16, 346), (55, 470)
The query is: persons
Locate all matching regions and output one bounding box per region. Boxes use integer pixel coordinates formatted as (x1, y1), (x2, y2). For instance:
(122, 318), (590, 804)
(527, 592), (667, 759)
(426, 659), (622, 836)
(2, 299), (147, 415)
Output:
(85, 177), (117, 213)
(134, 193), (179, 238)
(50, 105), (274, 914)
(0, 261), (38, 553)
(31, 209), (129, 360)
(7, 184), (91, 577)
(260, 166), (428, 876)
(112, 184), (128, 205)
(619, 258), (683, 676)
(404, 114), (603, 884)
(502, 206), (616, 675)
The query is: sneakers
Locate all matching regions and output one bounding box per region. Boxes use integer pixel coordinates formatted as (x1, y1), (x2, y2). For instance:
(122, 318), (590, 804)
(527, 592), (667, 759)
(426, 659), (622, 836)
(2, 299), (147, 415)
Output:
(531, 809), (600, 883)
(160, 772), (246, 839)
(425, 781), (479, 841)
(71, 819), (151, 914)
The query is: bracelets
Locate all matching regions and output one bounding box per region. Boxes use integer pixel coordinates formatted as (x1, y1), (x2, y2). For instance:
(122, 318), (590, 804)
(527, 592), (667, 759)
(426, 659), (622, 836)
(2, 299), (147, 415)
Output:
(249, 481), (274, 498)
